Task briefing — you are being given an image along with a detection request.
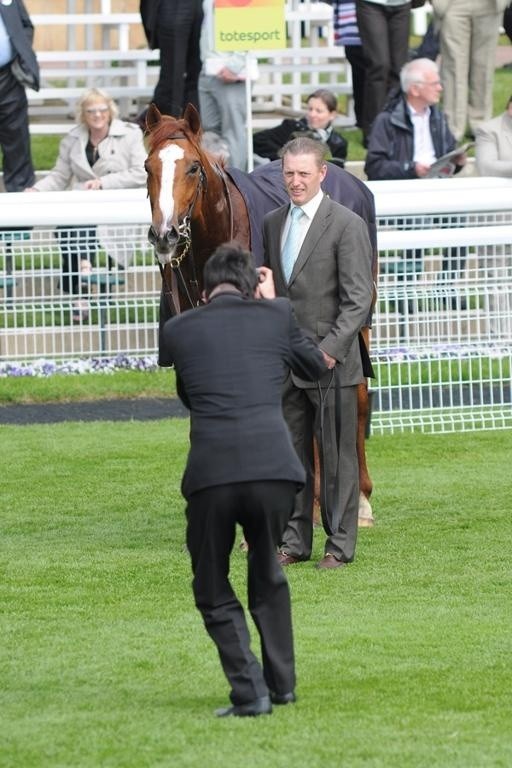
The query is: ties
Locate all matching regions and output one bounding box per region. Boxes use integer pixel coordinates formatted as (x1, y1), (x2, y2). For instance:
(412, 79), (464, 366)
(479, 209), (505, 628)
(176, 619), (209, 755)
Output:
(277, 207), (303, 282)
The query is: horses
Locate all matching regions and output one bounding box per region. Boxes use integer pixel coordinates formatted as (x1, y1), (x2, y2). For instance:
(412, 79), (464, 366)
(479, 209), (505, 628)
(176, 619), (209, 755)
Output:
(143, 99), (373, 529)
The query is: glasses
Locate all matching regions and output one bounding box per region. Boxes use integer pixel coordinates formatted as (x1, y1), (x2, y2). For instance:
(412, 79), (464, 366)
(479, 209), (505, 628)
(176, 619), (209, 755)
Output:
(83, 106), (107, 116)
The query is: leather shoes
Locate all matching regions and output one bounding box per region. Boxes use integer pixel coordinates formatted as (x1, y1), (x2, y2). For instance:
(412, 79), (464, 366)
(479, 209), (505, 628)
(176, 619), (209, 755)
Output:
(271, 547), (300, 562)
(215, 697), (270, 720)
(313, 554), (346, 572)
(266, 692), (293, 702)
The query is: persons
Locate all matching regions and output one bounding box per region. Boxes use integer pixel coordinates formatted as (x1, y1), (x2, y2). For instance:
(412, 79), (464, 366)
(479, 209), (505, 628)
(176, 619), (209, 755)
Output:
(260, 139), (375, 572)
(323, 1), (427, 148)
(165, 245), (329, 716)
(139, 0), (204, 119)
(432, 2), (507, 143)
(0, 0), (40, 192)
(23, 88), (150, 192)
(364, 58), (477, 180)
(475, 95), (511, 178)
(200, 2), (259, 173)
(254, 90), (348, 169)
(502, 2), (512, 59)
